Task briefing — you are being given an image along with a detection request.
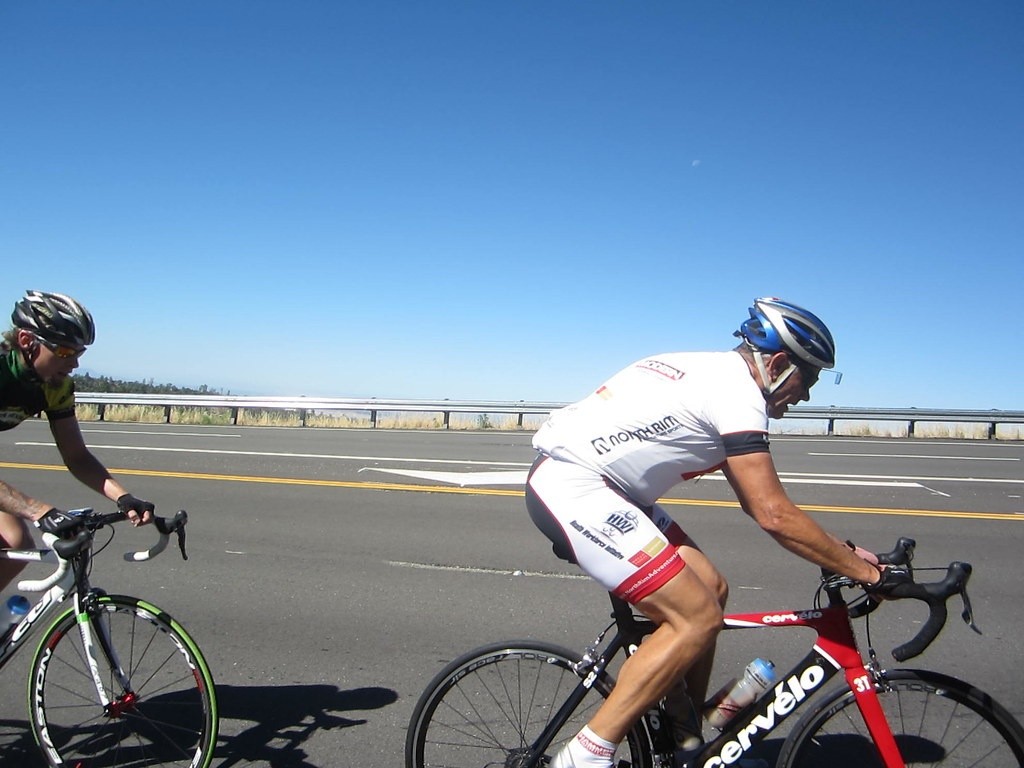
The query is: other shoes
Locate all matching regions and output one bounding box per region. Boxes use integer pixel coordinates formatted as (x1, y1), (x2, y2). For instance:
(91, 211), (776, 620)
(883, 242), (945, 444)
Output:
(549, 745), (626, 768)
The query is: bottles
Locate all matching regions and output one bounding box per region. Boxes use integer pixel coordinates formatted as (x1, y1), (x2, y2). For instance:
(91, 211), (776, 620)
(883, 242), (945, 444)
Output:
(702, 658), (777, 728)
(0, 595), (30, 640)
(660, 677), (704, 751)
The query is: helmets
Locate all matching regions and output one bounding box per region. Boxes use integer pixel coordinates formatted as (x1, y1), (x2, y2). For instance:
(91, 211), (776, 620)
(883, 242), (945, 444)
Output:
(12, 289), (96, 345)
(740, 297), (836, 368)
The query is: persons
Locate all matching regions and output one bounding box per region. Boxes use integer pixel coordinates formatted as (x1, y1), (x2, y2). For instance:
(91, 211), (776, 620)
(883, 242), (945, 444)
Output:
(0, 289), (155, 591)
(526, 298), (912, 768)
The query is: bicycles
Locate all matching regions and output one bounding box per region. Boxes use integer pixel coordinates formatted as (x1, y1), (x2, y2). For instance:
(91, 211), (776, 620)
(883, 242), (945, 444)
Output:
(402, 534), (1024, 767)
(0, 504), (221, 768)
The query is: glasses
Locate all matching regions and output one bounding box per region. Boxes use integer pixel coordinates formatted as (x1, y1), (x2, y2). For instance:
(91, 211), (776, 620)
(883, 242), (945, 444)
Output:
(26, 331), (86, 359)
(773, 350), (819, 389)
